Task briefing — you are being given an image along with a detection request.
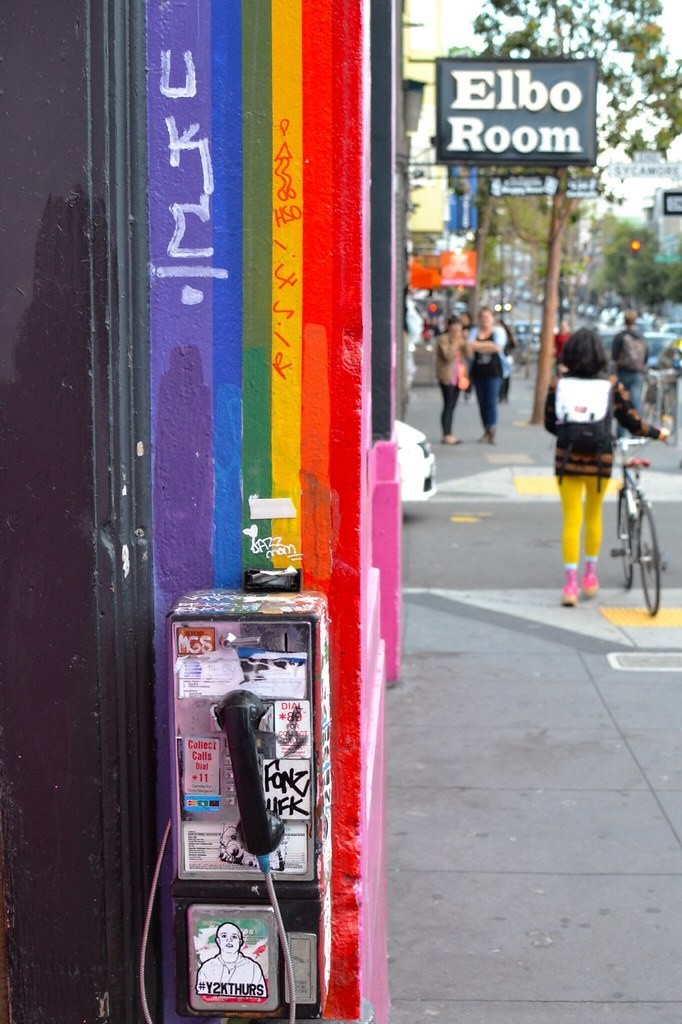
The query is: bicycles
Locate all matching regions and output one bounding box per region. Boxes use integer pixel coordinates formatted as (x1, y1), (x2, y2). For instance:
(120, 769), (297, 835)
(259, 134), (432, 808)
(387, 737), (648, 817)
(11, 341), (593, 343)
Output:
(610, 436), (668, 617)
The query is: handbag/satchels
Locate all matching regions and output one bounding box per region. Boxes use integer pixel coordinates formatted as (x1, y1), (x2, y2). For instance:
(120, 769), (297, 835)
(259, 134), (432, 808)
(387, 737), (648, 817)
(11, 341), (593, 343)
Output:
(458, 363), (470, 391)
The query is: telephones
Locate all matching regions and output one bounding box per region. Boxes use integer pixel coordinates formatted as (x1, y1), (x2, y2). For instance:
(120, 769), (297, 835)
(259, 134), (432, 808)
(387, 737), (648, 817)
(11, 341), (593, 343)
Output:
(159, 589), (332, 883)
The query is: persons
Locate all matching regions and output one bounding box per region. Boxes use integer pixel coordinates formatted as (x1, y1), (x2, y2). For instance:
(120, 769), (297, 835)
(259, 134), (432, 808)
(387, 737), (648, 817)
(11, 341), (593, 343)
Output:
(423, 316), (441, 341)
(467, 307), (514, 446)
(610, 310), (649, 436)
(435, 315), (465, 445)
(460, 312), (516, 405)
(555, 321), (572, 363)
(541, 328), (669, 605)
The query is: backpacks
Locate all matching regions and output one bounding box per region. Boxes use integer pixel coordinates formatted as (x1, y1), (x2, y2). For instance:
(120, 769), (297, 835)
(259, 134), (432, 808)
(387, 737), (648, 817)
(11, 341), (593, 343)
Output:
(554, 375), (613, 455)
(616, 329), (647, 373)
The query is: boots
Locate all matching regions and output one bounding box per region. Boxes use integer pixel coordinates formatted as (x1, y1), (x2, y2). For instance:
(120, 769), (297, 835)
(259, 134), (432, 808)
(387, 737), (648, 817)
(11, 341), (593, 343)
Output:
(561, 564), (579, 606)
(582, 557), (599, 598)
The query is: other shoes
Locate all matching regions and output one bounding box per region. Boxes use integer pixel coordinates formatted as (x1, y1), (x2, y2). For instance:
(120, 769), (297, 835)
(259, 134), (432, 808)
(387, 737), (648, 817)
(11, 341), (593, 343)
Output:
(441, 439), (462, 445)
(479, 429), (496, 445)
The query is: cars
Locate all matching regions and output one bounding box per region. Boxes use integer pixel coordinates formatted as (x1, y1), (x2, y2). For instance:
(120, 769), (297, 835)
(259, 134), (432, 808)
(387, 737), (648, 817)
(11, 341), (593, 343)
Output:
(504, 286), (682, 370)
(395, 418), (438, 503)
